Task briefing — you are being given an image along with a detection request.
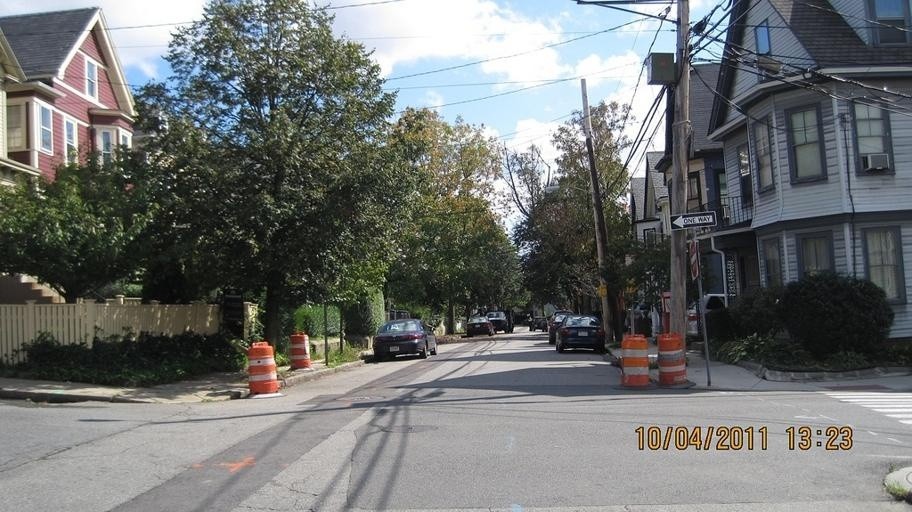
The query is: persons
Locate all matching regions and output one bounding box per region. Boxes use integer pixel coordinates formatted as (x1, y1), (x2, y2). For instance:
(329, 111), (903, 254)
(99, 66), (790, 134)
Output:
(528, 314), (533, 331)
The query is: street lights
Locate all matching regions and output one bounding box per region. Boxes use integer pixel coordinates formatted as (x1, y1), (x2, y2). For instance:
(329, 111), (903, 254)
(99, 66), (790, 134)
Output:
(544, 185), (614, 341)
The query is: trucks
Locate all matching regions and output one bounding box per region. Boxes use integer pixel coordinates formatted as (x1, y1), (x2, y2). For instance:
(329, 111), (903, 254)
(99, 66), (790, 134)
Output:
(485, 309), (515, 334)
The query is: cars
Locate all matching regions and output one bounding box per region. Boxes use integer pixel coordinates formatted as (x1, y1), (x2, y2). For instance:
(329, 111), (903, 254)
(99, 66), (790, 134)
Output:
(373, 318), (438, 359)
(627, 300), (651, 320)
(527, 310), (605, 355)
(684, 292), (735, 337)
(466, 316), (493, 337)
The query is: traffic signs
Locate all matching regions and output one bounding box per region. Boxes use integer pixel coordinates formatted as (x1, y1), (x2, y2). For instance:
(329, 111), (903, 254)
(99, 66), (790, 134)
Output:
(669, 210), (717, 231)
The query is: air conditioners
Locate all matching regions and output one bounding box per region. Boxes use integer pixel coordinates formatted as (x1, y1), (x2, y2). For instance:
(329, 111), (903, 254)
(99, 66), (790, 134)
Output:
(862, 153), (889, 171)
